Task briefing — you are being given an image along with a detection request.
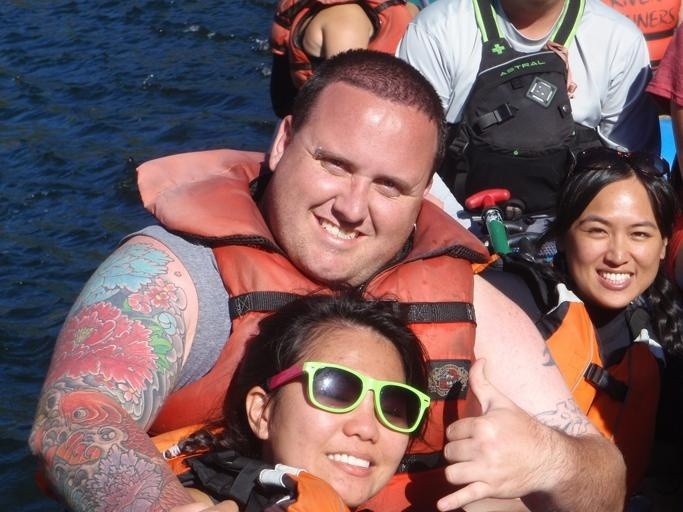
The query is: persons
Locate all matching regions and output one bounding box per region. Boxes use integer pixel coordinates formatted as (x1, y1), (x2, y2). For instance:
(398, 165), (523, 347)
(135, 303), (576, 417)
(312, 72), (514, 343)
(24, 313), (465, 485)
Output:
(167, 286), (532, 512)
(479, 150), (682, 512)
(270, 1), (421, 118)
(394, 1), (651, 275)
(29, 44), (633, 512)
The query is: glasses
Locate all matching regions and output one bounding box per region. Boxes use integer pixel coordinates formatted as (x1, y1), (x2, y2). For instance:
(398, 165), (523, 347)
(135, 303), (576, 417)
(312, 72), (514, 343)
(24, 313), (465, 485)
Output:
(572, 146), (670, 178)
(267, 361), (431, 435)
